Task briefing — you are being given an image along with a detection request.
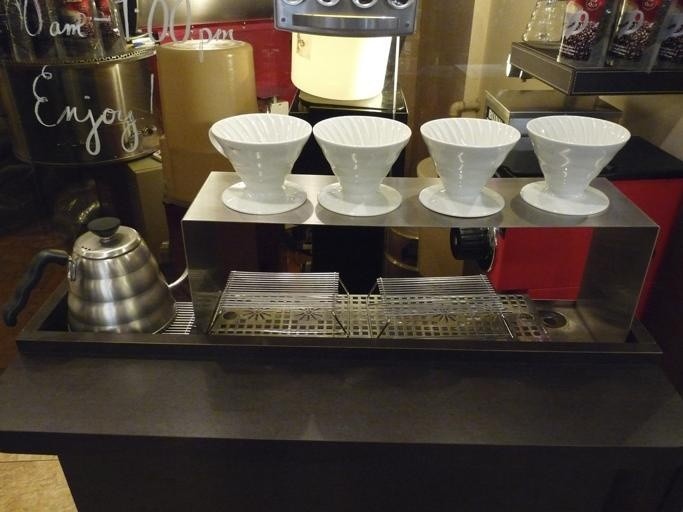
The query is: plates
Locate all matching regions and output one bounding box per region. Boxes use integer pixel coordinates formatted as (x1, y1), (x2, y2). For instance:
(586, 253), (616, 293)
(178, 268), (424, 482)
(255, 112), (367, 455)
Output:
(520, 179), (610, 218)
(220, 181), (309, 215)
(418, 183), (507, 218)
(317, 182), (403, 216)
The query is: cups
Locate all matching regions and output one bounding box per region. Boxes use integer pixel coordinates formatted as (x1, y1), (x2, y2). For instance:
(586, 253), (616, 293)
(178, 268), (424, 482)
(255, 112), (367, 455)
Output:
(525, 114), (631, 196)
(313, 115), (413, 195)
(419, 114), (523, 200)
(207, 113), (312, 195)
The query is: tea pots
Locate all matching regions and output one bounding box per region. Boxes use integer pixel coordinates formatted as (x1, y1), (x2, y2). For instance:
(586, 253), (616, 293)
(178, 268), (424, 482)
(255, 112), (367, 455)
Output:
(2, 217), (188, 339)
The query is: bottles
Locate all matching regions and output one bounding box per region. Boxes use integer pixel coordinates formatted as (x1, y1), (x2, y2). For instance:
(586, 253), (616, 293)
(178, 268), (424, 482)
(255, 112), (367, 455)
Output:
(522, 1), (683, 73)
